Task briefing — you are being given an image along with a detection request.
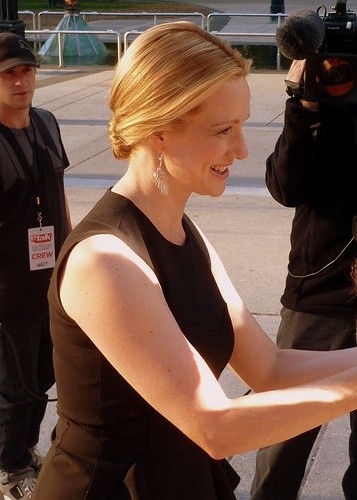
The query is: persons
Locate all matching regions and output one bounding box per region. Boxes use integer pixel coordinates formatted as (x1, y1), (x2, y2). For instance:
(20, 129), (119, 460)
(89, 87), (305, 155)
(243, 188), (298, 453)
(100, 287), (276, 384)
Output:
(31, 22), (357, 500)
(0, 33), (72, 500)
(250, 12), (357, 500)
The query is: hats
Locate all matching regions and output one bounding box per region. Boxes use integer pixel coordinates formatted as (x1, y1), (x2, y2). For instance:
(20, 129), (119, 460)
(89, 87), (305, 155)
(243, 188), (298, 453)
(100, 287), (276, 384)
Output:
(0, 32), (40, 73)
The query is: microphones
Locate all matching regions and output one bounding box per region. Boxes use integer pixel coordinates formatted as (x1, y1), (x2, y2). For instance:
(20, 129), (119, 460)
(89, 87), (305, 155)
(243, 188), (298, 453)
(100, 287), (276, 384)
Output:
(276, 8), (325, 60)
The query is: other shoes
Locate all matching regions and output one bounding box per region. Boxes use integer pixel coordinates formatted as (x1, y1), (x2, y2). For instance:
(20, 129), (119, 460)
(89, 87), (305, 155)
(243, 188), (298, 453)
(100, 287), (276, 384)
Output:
(1, 468), (37, 500)
(28, 452), (44, 478)
